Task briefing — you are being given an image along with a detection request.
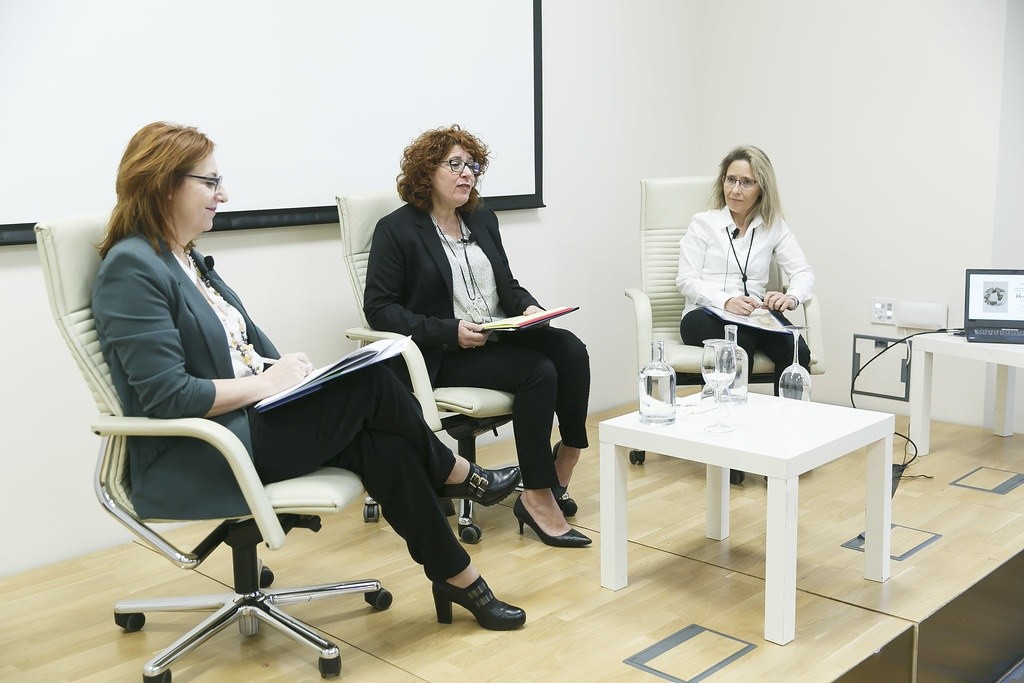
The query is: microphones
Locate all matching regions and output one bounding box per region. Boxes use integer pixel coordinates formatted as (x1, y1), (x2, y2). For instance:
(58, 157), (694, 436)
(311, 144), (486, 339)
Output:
(732, 228), (740, 239)
(204, 256), (215, 271)
(468, 233), (476, 244)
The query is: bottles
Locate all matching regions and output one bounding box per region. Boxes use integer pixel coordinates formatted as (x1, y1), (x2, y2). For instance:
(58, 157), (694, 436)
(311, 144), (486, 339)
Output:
(637, 341), (676, 425)
(714, 325), (748, 404)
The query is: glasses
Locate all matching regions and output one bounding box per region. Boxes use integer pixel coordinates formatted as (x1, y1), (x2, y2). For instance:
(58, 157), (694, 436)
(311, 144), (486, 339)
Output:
(722, 176), (758, 189)
(441, 159), (482, 175)
(183, 173), (224, 194)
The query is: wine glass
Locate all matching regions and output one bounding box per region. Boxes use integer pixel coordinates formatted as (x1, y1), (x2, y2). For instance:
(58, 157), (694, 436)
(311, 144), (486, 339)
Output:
(778, 326), (811, 404)
(701, 341), (737, 434)
(701, 339), (734, 403)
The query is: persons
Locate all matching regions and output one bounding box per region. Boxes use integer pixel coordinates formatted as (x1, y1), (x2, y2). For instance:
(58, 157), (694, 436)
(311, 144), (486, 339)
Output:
(365, 123), (592, 546)
(94, 122), (528, 629)
(675, 143), (815, 481)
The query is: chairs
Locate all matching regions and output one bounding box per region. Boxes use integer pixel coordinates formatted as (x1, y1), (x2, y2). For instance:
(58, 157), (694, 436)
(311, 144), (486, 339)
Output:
(33, 215), (391, 683)
(623, 175), (825, 486)
(335, 194), (526, 543)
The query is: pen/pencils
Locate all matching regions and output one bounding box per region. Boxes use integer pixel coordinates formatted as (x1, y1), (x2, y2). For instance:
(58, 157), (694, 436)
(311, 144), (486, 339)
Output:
(254, 357), (277, 365)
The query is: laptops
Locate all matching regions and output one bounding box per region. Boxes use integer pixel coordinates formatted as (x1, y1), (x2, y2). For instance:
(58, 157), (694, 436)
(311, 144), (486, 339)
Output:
(964, 268), (1024, 345)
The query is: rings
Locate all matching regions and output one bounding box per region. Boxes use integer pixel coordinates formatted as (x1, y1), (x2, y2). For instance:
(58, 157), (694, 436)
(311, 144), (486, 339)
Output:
(744, 310), (746, 313)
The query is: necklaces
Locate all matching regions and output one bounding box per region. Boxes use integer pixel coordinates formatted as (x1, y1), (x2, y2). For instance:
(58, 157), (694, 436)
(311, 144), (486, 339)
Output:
(726, 225), (755, 297)
(175, 248), (257, 376)
(436, 222), (487, 319)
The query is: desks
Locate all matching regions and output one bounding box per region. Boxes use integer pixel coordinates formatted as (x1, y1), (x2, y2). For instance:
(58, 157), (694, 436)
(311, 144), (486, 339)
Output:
(908, 333), (1024, 459)
(598, 390), (892, 647)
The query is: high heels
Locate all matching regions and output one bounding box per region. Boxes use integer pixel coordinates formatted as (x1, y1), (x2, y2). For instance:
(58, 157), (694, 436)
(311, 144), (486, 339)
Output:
(433, 575), (527, 631)
(436, 460), (521, 518)
(551, 439), (569, 500)
(513, 494), (592, 546)
